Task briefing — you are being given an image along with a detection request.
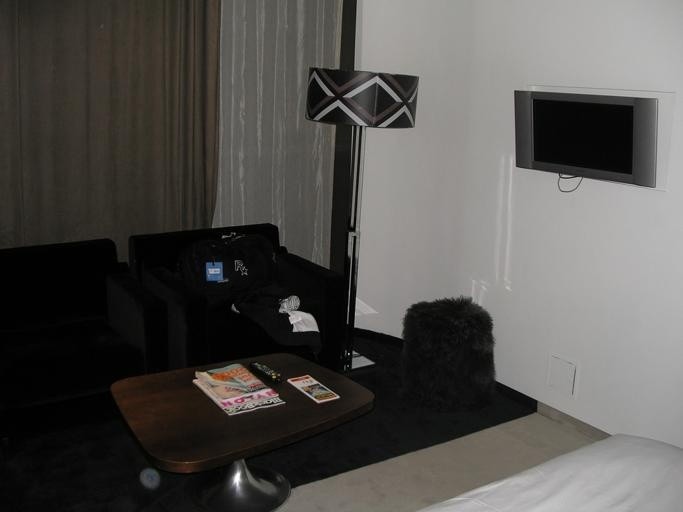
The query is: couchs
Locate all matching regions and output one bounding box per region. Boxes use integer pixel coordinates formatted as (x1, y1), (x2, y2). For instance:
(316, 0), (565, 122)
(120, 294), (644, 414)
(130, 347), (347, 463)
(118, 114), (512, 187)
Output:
(3, 238), (146, 406)
(122, 224), (346, 370)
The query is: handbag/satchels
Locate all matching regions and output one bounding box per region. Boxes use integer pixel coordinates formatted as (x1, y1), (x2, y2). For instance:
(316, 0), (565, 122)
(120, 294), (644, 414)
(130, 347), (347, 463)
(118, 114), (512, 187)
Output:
(180, 232), (280, 312)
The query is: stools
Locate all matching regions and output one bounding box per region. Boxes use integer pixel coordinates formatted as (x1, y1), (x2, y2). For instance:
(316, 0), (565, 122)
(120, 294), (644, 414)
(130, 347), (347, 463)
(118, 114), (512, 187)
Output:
(398, 296), (497, 410)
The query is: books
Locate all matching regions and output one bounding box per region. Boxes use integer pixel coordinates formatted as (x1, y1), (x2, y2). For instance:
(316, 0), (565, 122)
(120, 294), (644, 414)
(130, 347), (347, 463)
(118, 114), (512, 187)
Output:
(191, 362), (288, 416)
(287, 373), (341, 405)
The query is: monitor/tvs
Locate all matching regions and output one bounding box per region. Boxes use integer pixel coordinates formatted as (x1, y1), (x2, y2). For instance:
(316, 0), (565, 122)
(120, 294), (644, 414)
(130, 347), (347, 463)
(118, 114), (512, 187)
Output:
(515, 90), (658, 188)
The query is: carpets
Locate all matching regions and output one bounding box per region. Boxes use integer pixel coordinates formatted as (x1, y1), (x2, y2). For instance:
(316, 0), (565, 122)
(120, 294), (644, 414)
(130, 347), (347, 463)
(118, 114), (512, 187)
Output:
(0, 325), (538, 512)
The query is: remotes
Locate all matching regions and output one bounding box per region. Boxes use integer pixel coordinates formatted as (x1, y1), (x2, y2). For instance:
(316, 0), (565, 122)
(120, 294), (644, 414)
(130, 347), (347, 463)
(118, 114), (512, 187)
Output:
(250, 361), (287, 384)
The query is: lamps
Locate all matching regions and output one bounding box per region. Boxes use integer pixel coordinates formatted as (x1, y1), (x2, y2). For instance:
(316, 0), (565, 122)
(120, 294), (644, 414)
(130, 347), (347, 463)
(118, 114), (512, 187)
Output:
(304, 63), (419, 372)
